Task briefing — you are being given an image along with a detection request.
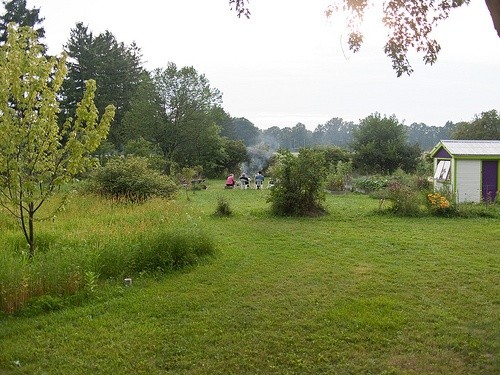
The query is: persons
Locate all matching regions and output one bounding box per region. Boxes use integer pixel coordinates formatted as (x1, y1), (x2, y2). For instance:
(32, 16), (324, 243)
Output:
(240, 173), (249, 188)
(255, 171), (264, 187)
(226, 174), (237, 188)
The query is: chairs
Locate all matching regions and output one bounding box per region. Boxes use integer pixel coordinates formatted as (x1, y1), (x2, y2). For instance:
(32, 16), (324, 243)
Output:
(240, 179), (250, 190)
(268, 178), (279, 189)
(254, 178), (263, 189)
